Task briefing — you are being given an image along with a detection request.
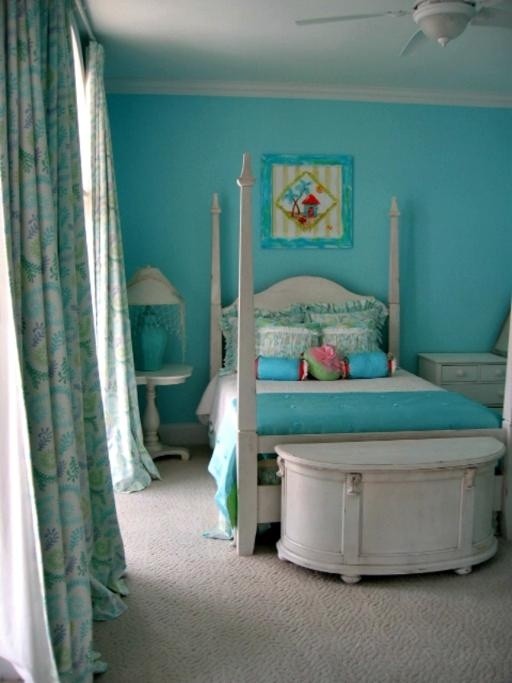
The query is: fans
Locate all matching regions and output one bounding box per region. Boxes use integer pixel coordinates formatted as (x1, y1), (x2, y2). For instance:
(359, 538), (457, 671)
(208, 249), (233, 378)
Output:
(296, 0), (512, 58)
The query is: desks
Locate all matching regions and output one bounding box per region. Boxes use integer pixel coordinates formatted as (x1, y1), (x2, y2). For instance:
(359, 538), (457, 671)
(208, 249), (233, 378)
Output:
(133, 366), (194, 466)
(274, 437), (506, 583)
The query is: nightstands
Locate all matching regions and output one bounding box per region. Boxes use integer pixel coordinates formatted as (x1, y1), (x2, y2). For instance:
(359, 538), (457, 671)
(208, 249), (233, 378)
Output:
(418, 350), (506, 418)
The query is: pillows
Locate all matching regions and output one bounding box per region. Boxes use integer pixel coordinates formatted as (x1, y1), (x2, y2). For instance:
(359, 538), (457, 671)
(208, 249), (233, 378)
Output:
(214, 301), (398, 381)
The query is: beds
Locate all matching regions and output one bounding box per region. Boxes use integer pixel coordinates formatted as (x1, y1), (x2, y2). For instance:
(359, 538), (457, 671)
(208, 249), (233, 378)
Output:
(195, 152), (512, 556)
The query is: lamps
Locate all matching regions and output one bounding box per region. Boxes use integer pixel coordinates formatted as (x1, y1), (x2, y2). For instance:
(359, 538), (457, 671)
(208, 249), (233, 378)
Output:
(413, 4), (473, 49)
(126, 265), (185, 371)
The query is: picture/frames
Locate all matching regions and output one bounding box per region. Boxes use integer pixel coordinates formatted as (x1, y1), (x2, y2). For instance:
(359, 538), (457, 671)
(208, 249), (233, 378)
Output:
(260, 153), (354, 249)
